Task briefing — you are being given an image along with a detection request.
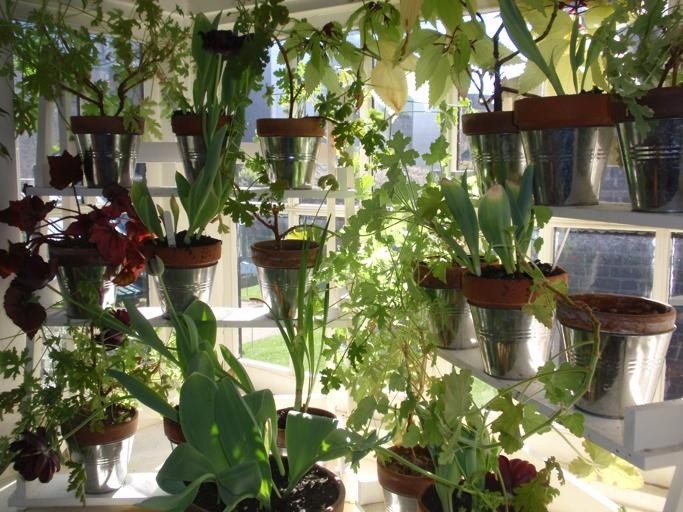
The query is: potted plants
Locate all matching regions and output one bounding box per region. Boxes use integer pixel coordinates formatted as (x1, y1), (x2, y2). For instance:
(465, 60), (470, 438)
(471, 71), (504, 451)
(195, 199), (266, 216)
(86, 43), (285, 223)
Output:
(1, 2), (683, 510)
